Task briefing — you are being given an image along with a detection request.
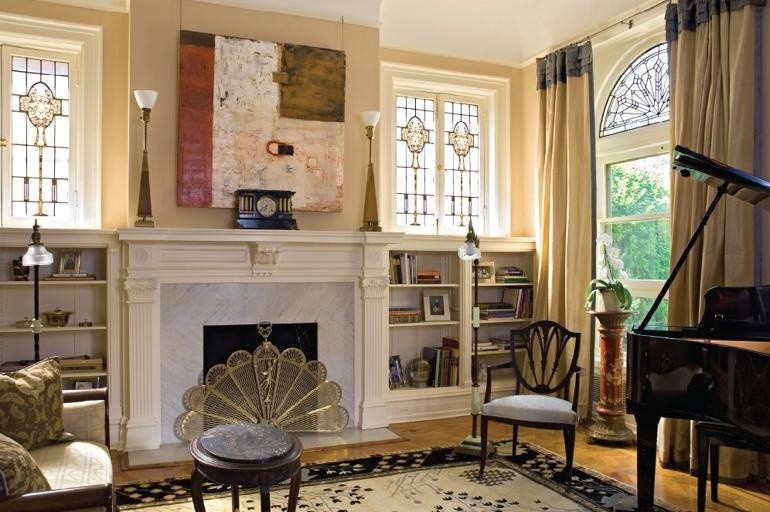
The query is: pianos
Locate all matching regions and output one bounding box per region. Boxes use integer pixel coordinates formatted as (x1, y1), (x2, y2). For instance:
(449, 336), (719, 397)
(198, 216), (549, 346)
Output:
(625, 145), (770, 512)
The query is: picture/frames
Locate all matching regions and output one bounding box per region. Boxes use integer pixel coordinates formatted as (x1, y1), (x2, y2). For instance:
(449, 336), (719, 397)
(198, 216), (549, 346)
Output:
(472, 261), (495, 283)
(59, 249), (80, 274)
(422, 288), (451, 321)
(58, 358), (103, 391)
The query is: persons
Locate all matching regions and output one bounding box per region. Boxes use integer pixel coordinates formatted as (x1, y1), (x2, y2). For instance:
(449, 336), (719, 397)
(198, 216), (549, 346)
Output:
(389, 363), (400, 384)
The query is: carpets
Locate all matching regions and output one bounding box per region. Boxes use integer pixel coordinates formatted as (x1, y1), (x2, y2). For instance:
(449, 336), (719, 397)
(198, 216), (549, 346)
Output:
(110, 438), (684, 512)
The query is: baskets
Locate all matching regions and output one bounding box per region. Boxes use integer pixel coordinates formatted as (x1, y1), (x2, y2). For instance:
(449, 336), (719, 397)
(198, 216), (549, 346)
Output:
(388, 307), (421, 323)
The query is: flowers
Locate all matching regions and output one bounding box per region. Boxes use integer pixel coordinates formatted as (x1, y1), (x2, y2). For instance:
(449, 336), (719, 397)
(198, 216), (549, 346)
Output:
(583, 234), (631, 309)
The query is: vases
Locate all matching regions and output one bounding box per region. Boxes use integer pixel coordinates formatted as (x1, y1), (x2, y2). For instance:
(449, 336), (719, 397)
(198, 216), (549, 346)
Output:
(598, 287), (622, 312)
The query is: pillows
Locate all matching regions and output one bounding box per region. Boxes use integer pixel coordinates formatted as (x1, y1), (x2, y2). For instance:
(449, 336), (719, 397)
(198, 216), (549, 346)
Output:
(0, 432), (52, 500)
(0, 355), (75, 452)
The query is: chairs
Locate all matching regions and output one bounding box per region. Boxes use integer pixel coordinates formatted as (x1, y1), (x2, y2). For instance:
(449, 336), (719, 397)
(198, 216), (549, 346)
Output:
(479, 321), (585, 493)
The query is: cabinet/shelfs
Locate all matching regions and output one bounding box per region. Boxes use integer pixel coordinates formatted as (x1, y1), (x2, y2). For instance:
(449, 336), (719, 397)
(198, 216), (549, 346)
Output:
(467, 238), (536, 415)
(0, 228), (109, 388)
(387, 237), (467, 425)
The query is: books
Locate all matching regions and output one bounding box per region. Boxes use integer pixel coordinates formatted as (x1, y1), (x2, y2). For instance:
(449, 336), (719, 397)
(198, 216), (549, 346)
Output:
(472, 338), (527, 351)
(389, 252), (441, 284)
(495, 266), (530, 283)
(423, 337), (460, 388)
(478, 288), (533, 321)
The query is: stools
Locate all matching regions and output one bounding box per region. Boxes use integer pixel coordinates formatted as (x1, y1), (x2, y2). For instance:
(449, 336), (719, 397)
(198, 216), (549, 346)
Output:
(189, 423), (302, 512)
(694, 418), (770, 512)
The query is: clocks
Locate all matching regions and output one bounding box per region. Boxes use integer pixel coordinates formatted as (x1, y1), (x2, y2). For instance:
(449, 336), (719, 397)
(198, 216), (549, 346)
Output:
(234, 189), (298, 229)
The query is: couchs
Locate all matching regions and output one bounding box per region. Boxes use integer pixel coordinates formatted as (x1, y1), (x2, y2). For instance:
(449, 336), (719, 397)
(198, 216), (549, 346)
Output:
(0, 388), (116, 512)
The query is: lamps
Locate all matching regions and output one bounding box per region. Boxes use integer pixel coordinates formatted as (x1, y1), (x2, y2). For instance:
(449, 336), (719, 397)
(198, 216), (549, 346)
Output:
(134, 89), (158, 227)
(457, 222), (495, 458)
(22, 219), (54, 361)
(360, 111), (382, 232)
